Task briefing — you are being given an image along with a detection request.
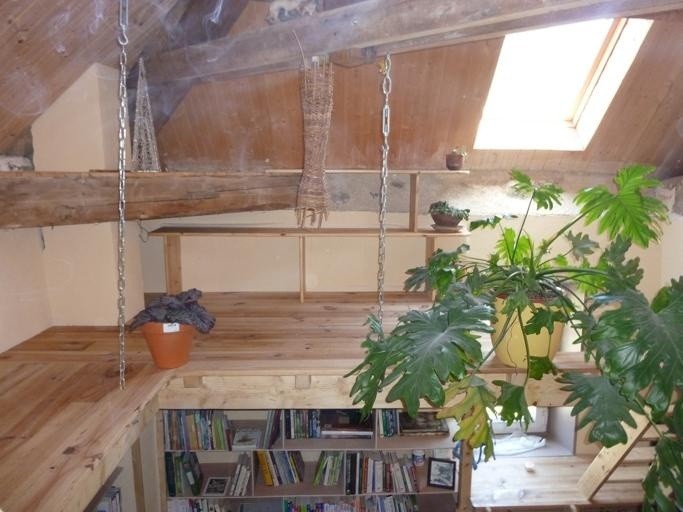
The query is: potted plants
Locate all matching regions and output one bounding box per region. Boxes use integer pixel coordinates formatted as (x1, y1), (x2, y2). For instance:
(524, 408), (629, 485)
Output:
(128, 288), (215, 370)
(429, 200), (470, 232)
(446, 146), (468, 169)
(343, 169), (683, 512)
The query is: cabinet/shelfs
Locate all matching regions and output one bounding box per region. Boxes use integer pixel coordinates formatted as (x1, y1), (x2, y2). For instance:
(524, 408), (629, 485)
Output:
(93, 428), (148, 510)
(156, 396), (461, 508)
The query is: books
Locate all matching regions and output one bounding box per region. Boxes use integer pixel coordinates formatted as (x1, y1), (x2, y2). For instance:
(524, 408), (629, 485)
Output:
(90, 488), (120, 510)
(161, 408), (451, 511)
